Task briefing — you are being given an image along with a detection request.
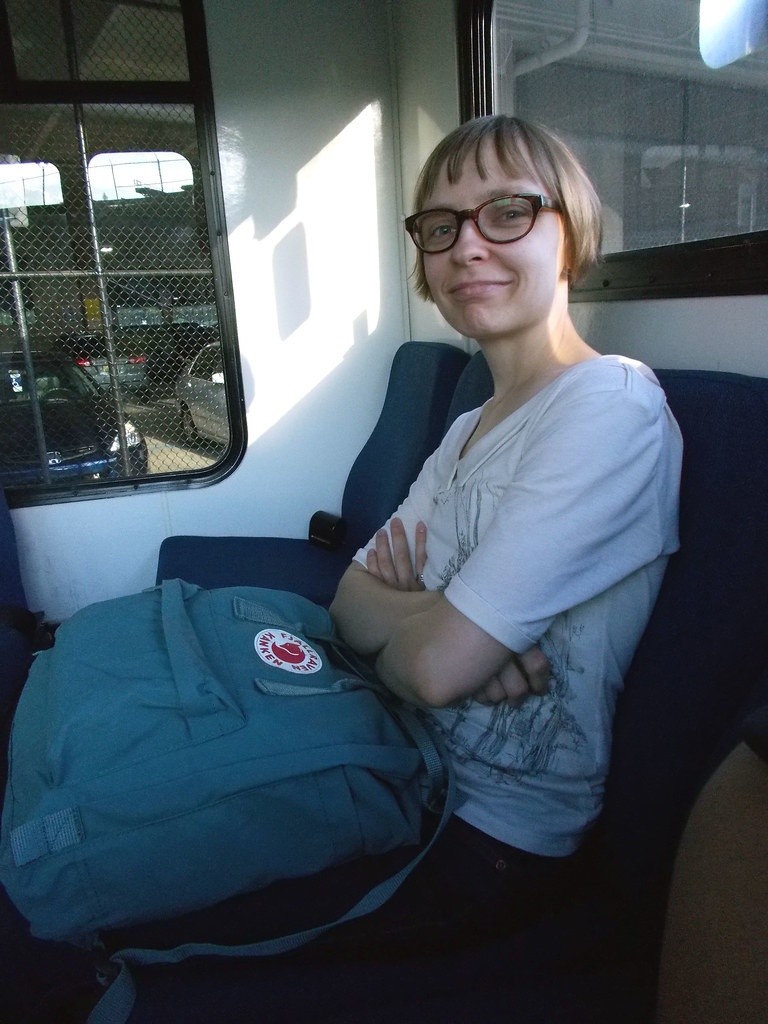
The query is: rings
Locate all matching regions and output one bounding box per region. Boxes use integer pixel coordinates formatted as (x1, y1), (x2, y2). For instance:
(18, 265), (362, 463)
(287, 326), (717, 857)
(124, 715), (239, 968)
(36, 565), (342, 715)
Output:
(415, 574), (425, 583)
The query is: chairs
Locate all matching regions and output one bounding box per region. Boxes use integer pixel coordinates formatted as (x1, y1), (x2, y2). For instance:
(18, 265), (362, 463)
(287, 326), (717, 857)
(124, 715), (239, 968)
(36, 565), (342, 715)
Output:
(17, 377), (62, 401)
(157, 341), (767, 1024)
(0, 492), (45, 764)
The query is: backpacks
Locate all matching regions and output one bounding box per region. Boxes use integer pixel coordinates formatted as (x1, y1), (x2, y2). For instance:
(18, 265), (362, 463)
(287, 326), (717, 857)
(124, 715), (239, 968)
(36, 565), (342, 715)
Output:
(0, 578), (458, 1023)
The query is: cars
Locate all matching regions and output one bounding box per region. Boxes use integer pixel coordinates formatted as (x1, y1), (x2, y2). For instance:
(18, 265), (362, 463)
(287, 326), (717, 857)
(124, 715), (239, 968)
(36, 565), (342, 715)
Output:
(172, 342), (230, 450)
(0, 340), (148, 491)
(56, 334), (154, 405)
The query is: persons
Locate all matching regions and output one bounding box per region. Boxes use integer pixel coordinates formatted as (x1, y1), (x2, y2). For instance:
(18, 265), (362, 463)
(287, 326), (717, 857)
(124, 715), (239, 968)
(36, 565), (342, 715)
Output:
(1, 112), (683, 1024)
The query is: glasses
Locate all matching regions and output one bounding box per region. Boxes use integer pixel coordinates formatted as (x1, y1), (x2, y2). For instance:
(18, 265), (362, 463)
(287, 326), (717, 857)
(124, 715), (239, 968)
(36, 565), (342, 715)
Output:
(403, 193), (562, 255)
